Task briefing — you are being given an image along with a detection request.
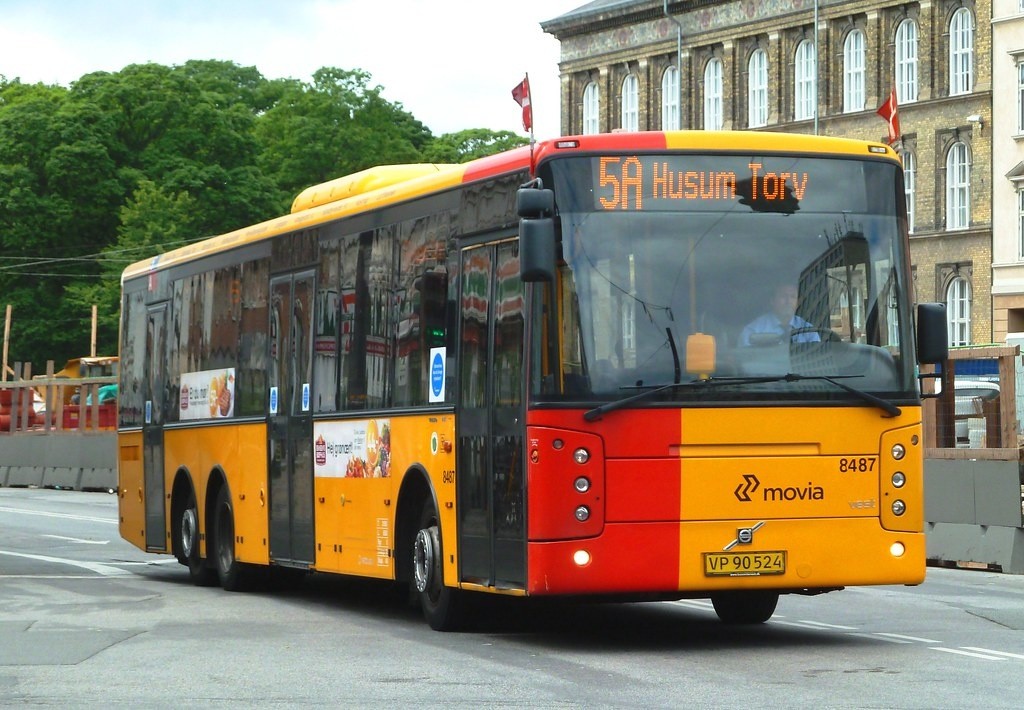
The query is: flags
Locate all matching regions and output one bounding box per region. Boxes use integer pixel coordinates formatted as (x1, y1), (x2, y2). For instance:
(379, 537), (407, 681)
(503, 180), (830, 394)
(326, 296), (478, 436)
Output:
(511, 75), (531, 132)
(876, 88), (899, 145)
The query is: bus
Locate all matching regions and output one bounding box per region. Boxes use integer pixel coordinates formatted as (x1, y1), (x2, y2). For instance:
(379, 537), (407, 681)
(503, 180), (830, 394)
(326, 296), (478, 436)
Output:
(115, 130), (927, 634)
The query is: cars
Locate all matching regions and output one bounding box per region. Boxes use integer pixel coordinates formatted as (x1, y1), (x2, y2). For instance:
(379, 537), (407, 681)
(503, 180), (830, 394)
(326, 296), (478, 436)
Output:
(916, 378), (1000, 450)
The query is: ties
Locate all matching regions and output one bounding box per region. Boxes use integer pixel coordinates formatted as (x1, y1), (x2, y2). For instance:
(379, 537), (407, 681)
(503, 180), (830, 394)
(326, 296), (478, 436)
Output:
(780, 324), (793, 343)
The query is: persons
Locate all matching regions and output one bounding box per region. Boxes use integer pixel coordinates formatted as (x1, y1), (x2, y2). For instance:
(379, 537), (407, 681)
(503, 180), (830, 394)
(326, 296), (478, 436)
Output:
(71, 387), (81, 405)
(737, 281), (821, 348)
